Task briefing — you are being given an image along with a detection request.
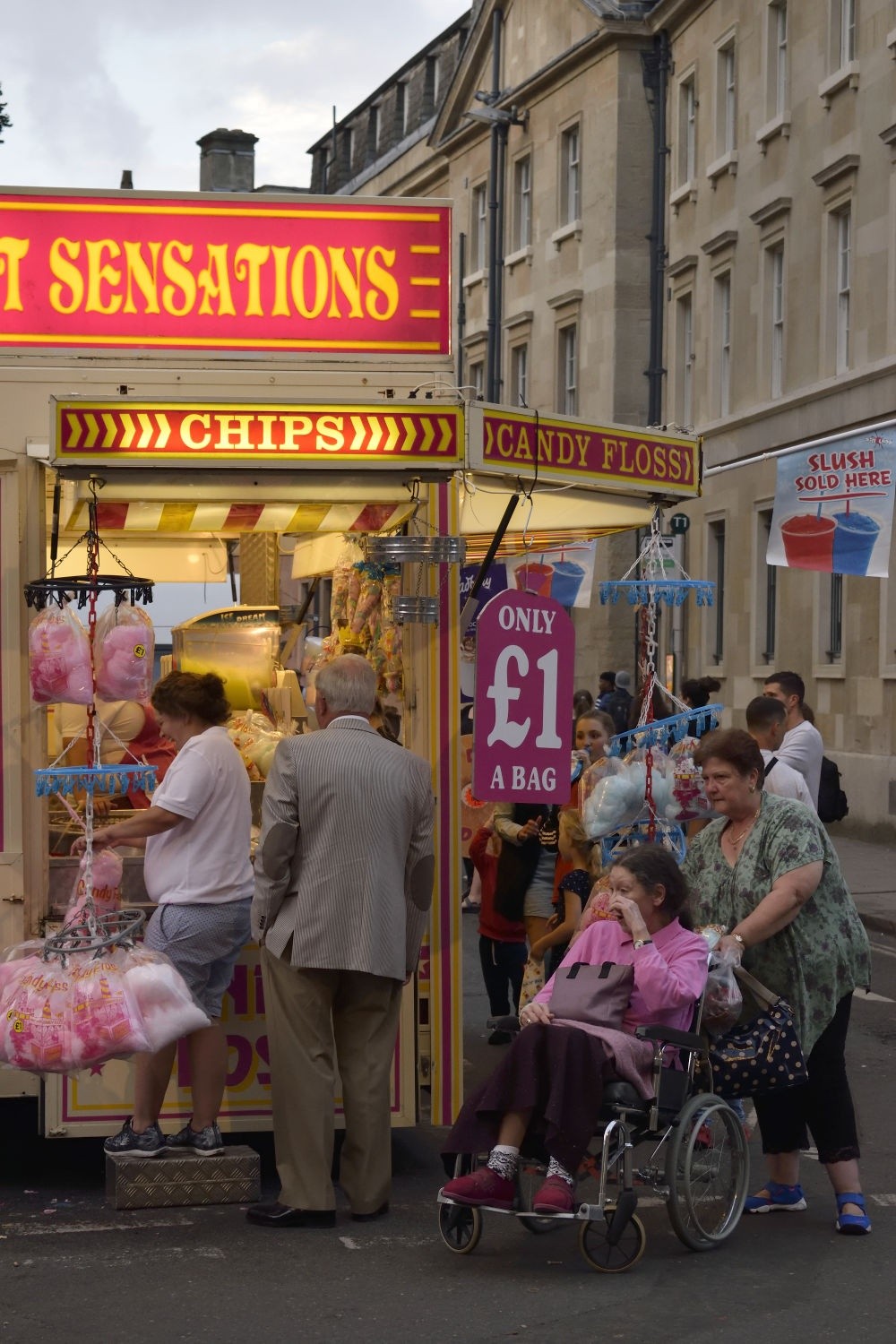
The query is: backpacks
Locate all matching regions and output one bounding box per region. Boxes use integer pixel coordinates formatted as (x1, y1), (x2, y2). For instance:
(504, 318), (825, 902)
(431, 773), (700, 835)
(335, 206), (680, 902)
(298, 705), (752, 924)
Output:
(817, 755), (849, 824)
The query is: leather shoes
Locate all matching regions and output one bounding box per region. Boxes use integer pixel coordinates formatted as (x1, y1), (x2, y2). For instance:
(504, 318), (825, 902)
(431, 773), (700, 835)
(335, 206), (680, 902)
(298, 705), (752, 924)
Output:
(246, 1201), (337, 1228)
(352, 1200), (391, 1222)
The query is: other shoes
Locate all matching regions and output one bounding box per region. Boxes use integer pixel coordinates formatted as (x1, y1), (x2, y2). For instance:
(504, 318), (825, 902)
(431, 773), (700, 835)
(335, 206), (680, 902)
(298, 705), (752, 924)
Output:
(692, 1124), (715, 1149)
(487, 1029), (517, 1045)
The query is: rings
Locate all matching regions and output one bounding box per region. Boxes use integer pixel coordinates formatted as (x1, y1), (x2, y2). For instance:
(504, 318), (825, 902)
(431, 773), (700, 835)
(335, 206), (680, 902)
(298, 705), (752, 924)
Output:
(526, 1019), (530, 1024)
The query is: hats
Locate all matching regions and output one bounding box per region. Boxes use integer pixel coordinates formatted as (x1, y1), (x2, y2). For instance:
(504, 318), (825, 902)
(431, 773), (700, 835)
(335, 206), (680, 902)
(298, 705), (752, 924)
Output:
(614, 670), (632, 689)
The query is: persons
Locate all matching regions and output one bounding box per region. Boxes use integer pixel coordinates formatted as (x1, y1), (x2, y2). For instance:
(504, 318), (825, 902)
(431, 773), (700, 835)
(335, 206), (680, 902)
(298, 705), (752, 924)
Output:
(68, 670), (256, 1157)
(456, 669), (720, 1029)
(440, 842), (711, 1212)
(682, 1086), (752, 1153)
(763, 671), (823, 812)
(679, 726), (872, 1235)
(245, 654), (436, 1228)
(746, 696), (817, 818)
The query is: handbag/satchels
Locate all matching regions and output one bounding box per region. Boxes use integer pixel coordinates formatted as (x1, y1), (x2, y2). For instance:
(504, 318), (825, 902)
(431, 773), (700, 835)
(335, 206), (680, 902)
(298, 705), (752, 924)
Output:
(93, 591), (155, 708)
(62, 842), (124, 938)
(0, 939), (217, 1083)
(28, 590), (94, 712)
(693, 923), (745, 1038)
(707, 964), (809, 1101)
(227, 708), (297, 780)
(549, 962), (634, 1032)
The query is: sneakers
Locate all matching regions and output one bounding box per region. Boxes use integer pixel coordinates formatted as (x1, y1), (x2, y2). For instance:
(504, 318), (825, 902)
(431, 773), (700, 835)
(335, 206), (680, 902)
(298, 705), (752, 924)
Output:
(442, 1166), (515, 1209)
(166, 1118), (225, 1157)
(103, 1116), (168, 1157)
(534, 1174), (575, 1214)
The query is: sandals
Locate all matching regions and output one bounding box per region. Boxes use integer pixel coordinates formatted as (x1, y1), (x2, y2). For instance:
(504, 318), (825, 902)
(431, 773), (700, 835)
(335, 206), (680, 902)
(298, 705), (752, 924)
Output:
(835, 1192), (871, 1234)
(461, 897), (481, 913)
(741, 1182), (807, 1213)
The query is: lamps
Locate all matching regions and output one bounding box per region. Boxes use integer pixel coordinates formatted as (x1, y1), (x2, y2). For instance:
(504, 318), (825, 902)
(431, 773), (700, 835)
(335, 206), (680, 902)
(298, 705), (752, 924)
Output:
(461, 103), (531, 134)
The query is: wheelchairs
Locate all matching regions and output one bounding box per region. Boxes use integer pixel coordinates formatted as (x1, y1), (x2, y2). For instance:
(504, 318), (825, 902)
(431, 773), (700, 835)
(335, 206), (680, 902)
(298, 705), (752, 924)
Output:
(439, 933), (759, 1274)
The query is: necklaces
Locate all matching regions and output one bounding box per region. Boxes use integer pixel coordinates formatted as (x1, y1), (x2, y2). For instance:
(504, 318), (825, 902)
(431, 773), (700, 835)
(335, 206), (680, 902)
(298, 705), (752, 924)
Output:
(727, 809), (762, 844)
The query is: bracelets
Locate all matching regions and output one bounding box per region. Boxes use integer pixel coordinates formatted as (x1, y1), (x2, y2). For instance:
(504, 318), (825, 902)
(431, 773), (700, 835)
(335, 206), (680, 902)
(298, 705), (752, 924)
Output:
(732, 933), (746, 947)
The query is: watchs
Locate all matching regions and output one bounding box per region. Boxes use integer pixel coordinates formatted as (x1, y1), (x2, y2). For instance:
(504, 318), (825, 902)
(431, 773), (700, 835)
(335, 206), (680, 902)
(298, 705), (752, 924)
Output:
(633, 939), (653, 950)
